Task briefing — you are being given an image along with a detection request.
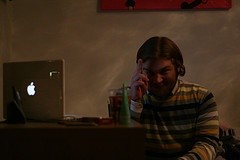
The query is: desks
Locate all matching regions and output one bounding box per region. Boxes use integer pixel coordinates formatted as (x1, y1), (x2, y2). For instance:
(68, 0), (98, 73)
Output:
(0, 120), (147, 160)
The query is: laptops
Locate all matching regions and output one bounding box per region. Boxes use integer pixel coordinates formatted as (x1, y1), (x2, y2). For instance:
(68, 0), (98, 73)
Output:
(3, 59), (103, 121)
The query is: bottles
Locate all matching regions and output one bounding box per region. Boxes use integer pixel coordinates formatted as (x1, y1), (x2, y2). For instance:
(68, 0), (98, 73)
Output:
(119, 88), (131, 125)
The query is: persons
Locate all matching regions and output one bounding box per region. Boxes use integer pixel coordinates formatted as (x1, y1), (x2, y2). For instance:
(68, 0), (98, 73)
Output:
(127, 37), (219, 160)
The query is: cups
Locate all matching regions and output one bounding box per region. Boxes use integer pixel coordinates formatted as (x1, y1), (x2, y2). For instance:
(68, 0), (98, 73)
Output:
(110, 96), (131, 122)
